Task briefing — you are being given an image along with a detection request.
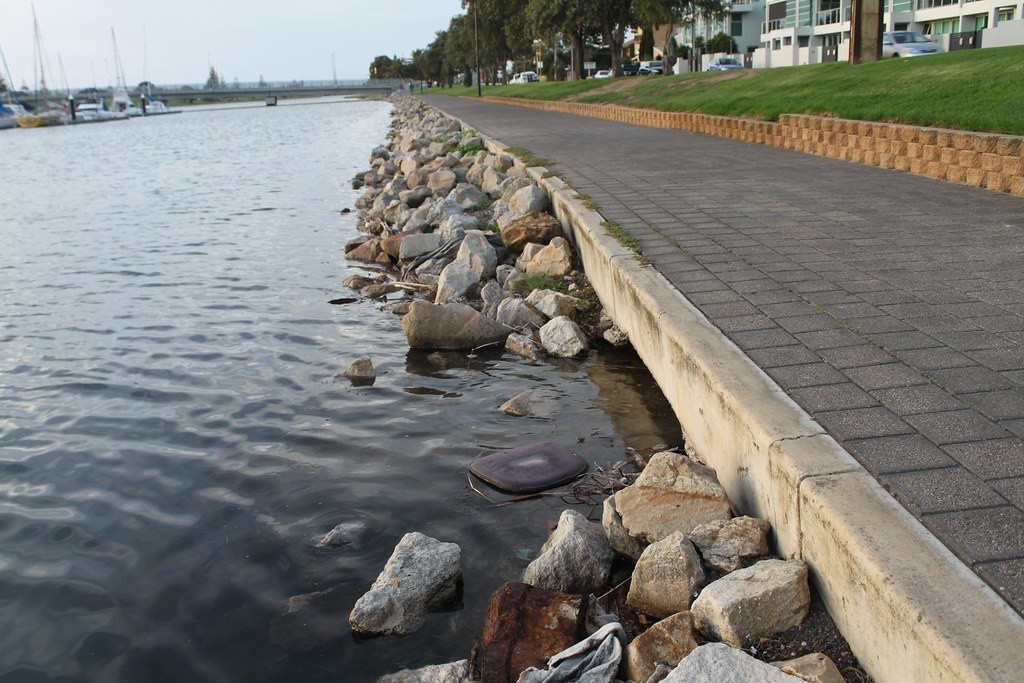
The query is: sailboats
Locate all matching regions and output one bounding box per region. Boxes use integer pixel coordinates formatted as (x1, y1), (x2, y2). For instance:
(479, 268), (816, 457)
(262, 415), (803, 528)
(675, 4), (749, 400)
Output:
(0, 0), (183, 130)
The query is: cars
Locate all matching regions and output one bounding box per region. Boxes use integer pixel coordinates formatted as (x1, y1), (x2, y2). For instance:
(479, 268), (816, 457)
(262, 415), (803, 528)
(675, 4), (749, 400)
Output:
(510, 71), (539, 84)
(882, 30), (942, 59)
(595, 71), (610, 79)
(637, 61), (674, 76)
(609, 64), (638, 76)
(707, 58), (745, 71)
